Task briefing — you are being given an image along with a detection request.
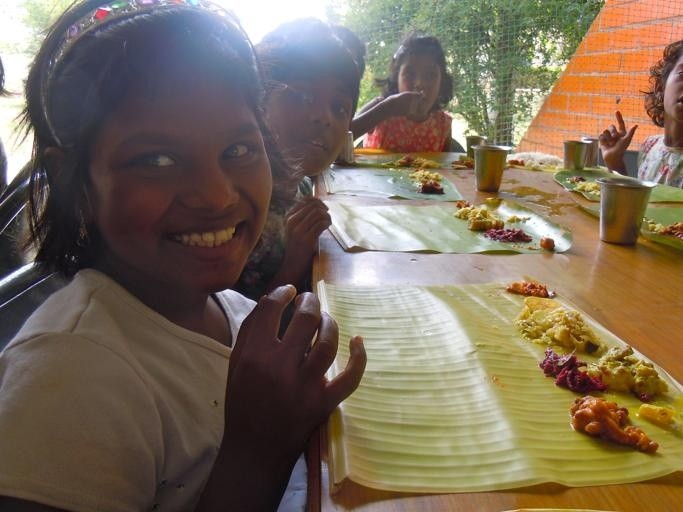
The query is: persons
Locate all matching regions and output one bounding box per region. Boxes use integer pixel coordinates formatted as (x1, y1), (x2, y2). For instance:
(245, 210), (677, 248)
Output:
(598, 36), (683, 192)
(230, 16), (362, 305)
(332, 22), (366, 77)
(0, 61), (23, 194)
(0, 1), (366, 511)
(349, 31), (455, 151)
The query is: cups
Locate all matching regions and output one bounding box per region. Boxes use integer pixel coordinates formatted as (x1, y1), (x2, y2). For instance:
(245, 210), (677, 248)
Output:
(465, 135), (487, 158)
(470, 145), (512, 192)
(595, 177), (657, 246)
(581, 138), (598, 168)
(560, 140), (593, 170)
